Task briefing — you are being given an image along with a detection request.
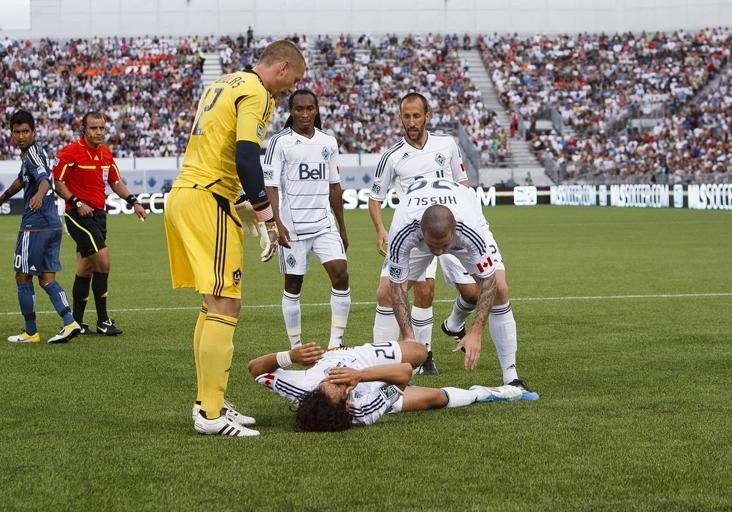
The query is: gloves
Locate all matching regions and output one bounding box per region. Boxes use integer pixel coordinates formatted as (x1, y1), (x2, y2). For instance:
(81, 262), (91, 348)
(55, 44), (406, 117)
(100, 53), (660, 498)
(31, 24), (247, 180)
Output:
(251, 202), (280, 261)
(233, 194), (259, 236)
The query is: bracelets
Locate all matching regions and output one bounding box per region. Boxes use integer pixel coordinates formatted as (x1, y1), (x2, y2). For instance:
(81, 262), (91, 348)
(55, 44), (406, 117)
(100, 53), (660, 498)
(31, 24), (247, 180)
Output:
(68, 195), (80, 207)
(126, 193), (137, 206)
(275, 349), (293, 370)
(133, 201), (142, 205)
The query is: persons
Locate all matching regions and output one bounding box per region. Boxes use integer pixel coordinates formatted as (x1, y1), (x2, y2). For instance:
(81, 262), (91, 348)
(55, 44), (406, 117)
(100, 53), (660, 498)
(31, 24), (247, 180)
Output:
(2, 109), (83, 345)
(367, 95), (482, 376)
(51, 112), (149, 338)
(243, 340), (524, 432)
(371, 176), (541, 406)
(165, 37), (307, 438)
(262, 90), (353, 351)
(1, 24), (731, 194)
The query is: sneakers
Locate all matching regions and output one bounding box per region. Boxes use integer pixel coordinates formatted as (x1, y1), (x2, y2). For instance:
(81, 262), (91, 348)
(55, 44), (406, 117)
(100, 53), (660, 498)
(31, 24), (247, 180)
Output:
(508, 379), (540, 400)
(96, 318), (123, 336)
(47, 320), (96, 343)
(7, 328), (41, 343)
(192, 399), (259, 437)
(422, 350), (439, 375)
(441, 319), (465, 352)
(469, 385), (522, 403)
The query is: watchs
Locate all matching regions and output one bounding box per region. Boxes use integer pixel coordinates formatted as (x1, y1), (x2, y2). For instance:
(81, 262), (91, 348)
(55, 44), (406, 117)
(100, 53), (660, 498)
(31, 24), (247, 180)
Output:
(74, 200), (83, 209)
